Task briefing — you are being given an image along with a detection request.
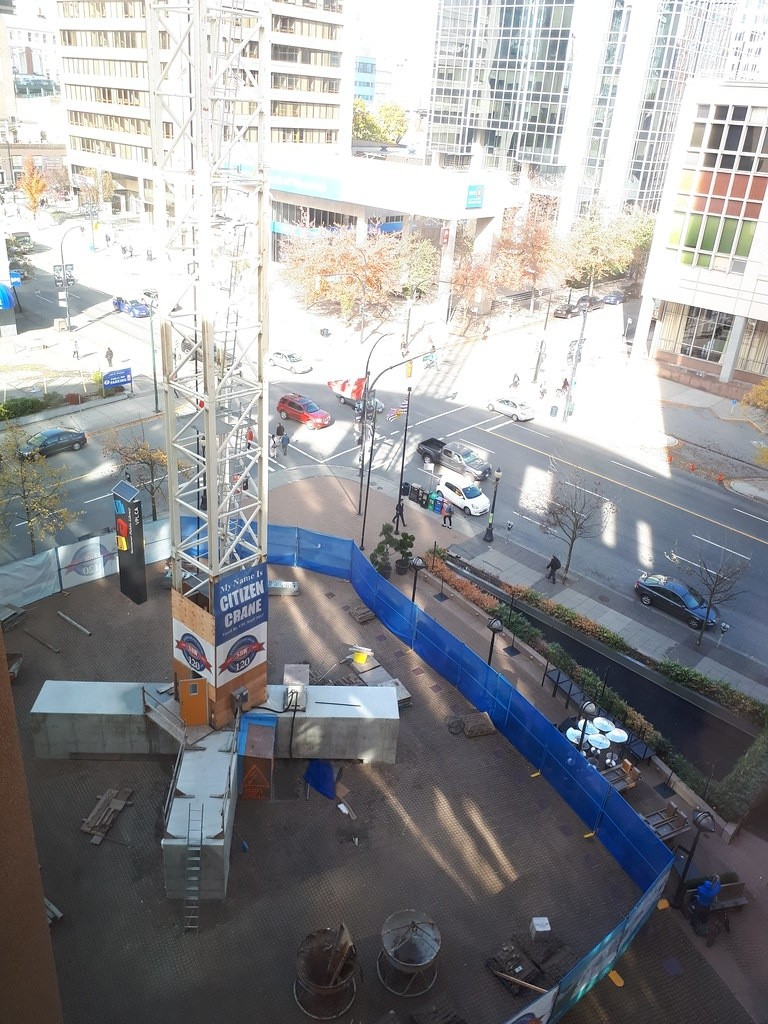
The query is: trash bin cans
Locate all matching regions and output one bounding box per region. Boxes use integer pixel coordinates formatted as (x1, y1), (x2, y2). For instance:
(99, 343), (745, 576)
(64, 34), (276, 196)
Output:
(550, 406), (558, 417)
(232, 472), (248, 493)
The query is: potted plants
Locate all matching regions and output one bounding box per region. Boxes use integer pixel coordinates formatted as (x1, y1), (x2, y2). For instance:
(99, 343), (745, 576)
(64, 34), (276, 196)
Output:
(370, 523), (415, 579)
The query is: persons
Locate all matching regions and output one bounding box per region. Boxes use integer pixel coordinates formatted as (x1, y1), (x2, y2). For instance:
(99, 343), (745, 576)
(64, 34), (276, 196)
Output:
(105, 347), (113, 367)
(691, 875), (721, 926)
(245, 428), (253, 450)
(73, 340), (80, 361)
(392, 499), (407, 527)
(105, 232), (152, 262)
(199, 398), (204, 408)
(270, 423), (290, 459)
(423, 345), (441, 371)
(482, 324), (490, 341)
(546, 554), (561, 584)
(510, 373), (569, 398)
(441, 499), (452, 530)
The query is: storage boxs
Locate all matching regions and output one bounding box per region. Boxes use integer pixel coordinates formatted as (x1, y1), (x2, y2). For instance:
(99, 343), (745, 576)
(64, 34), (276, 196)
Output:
(530, 917), (551, 942)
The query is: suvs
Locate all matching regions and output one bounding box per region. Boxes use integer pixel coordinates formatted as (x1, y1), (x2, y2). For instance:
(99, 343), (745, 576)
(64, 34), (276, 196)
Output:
(277, 393), (331, 430)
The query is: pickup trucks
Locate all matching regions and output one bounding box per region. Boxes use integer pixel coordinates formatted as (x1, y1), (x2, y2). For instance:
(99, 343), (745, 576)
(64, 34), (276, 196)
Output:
(416, 438), (492, 481)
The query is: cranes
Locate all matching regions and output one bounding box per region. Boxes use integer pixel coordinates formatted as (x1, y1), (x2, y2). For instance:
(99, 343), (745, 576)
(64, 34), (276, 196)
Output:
(144, 1), (269, 731)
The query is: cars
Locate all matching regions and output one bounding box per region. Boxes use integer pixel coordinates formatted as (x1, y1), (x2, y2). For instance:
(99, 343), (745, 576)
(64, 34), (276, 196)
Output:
(436, 474), (490, 516)
(113, 297), (216, 365)
(634, 573), (722, 630)
(16, 427), (87, 463)
(554, 285), (643, 319)
(486, 398), (535, 421)
(268, 349), (384, 414)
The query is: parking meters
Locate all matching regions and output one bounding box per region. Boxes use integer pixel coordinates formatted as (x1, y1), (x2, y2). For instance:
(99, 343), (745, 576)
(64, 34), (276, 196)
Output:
(715, 623), (730, 648)
(506, 520), (513, 543)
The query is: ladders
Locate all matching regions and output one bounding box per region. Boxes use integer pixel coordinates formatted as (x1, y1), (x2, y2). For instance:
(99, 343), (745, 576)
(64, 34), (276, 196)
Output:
(183, 802), (204, 936)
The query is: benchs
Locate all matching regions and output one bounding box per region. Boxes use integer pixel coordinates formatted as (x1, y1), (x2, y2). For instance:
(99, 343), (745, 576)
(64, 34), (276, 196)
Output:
(681, 882), (750, 920)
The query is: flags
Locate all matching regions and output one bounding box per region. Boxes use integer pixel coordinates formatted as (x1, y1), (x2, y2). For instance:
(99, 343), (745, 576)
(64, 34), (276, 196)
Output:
(386, 397), (408, 421)
(327, 378), (365, 402)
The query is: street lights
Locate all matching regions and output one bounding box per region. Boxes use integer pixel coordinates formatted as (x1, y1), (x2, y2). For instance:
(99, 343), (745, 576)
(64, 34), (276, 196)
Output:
(526, 269), (551, 382)
(565, 698), (598, 789)
(408, 556), (427, 630)
(483, 467), (502, 543)
(200, 436), (207, 511)
(671, 808), (716, 910)
(61, 226), (85, 332)
(483, 617), (504, 696)
